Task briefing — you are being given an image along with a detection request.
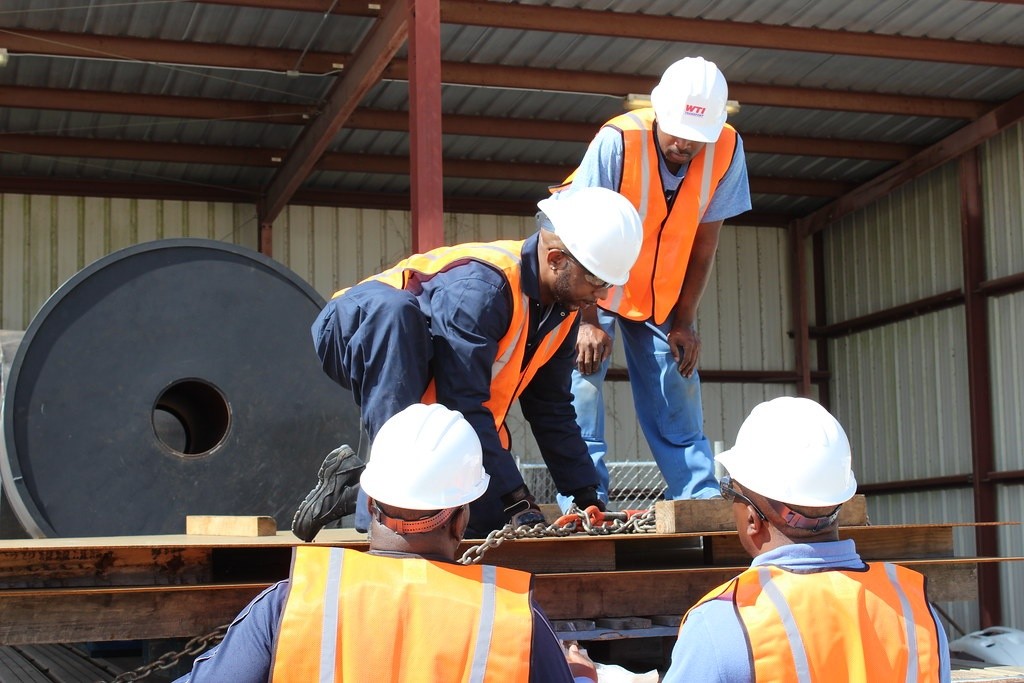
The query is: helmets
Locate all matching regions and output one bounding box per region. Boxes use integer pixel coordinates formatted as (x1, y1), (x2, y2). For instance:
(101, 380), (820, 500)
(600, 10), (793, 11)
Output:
(537, 187), (643, 286)
(714, 396), (857, 507)
(360, 403), (491, 510)
(651, 56), (728, 143)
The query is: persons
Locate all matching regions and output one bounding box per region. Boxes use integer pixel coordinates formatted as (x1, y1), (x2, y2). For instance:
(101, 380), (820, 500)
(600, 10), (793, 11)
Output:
(662, 395), (952, 683)
(292, 185), (643, 543)
(179, 402), (597, 683)
(548, 57), (751, 513)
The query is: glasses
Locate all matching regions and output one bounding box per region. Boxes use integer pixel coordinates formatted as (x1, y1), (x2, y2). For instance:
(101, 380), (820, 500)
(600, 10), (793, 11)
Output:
(548, 248), (614, 289)
(720, 476), (767, 521)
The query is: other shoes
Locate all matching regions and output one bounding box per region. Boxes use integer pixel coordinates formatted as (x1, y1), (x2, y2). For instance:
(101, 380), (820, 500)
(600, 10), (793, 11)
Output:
(292, 444), (366, 543)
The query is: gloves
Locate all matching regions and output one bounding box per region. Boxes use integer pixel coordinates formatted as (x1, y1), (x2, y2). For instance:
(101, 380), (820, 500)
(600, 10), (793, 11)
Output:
(501, 483), (541, 515)
